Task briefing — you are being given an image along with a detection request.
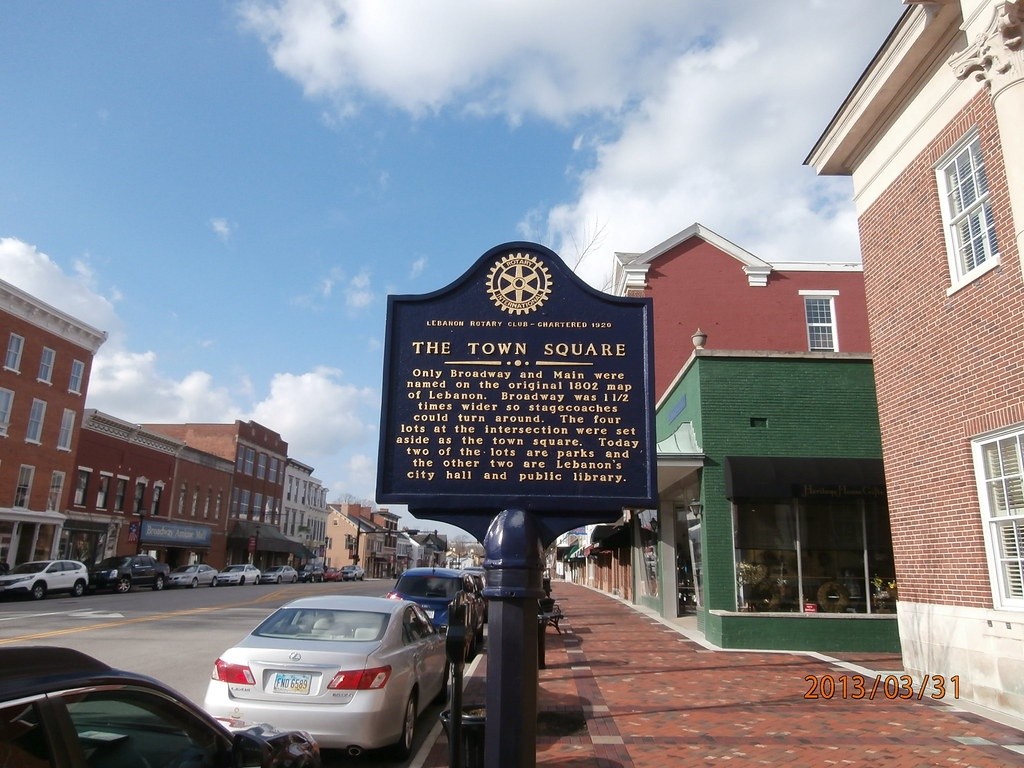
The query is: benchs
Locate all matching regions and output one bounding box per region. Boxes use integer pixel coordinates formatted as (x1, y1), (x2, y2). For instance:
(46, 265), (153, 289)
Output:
(542, 605), (564, 635)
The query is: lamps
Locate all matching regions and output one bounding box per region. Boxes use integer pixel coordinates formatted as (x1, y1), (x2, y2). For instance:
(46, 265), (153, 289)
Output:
(688, 498), (703, 518)
(650, 518), (660, 532)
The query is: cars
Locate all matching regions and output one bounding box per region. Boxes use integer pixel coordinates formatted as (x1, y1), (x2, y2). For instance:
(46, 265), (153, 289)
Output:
(169, 564), (218, 588)
(0, 646), (321, 768)
(386, 566), (488, 663)
(340, 565), (365, 582)
(0, 560), (89, 600)
(260, 565), (298, 584)
(204, 595), (456, 761)
(89, 554), (171, 593)
(218, 564), (262, 586)
(324, 568), (343, 582)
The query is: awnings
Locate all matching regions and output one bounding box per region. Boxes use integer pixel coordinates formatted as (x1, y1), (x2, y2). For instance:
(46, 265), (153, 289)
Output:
(230, 521), (317, 558)
(376, 554), (413, 561)
(555, 451), (702, 561)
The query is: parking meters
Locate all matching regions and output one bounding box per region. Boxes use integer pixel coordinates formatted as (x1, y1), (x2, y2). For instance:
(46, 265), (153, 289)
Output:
(445, 591), (466, 768)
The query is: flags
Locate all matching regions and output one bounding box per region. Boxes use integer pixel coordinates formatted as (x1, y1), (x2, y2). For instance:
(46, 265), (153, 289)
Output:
(128, 523), (137, 542)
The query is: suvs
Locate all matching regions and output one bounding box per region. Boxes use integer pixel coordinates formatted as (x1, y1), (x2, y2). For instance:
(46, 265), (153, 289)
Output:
(295, 563), (325, 583)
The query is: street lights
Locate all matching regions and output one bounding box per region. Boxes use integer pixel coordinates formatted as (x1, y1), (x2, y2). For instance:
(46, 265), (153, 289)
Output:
(254, 523), (261, 567)
(323, 535), (330, 568)
(136, 506), (147, 555)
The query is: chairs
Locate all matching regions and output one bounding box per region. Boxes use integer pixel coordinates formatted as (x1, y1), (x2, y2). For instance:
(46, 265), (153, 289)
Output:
(278, 624), (307, 635)
(355, 628), (377, 639)
